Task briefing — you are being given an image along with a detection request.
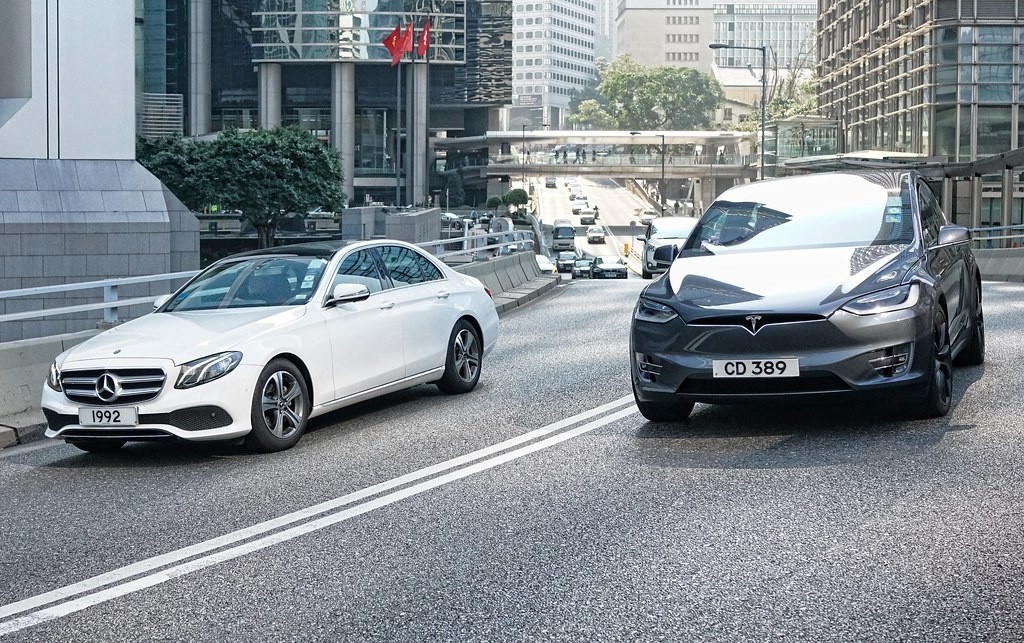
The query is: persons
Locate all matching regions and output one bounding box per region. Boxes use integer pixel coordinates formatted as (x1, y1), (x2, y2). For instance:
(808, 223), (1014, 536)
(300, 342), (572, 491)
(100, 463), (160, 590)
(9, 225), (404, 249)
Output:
(470, 209), (478, 224)
(582, 149), (587, 164)
(592, 150), (596, 164)
(694, 151), (698, 164)
(572, 148), (581, 164)
(719, 150), (727, 165)
(525, 150), (532, 164)
(630, 150), (636, 164)
(555, 150), (560, 164)
(800, 133), (817, 155)
(563, 151), (568, 164)
(668, 152), (673, 164)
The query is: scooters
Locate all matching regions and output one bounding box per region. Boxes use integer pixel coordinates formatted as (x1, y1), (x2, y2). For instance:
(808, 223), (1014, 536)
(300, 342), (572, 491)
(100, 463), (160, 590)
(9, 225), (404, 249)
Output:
(594, 210), (599, 218)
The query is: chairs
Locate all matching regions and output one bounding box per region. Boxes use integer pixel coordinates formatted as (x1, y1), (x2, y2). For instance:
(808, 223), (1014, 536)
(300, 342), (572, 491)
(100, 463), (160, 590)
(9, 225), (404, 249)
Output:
(250, 267), (290, 303)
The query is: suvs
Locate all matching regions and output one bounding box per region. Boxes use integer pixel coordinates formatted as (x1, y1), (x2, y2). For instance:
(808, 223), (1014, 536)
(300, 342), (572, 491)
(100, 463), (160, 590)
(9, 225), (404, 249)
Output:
(308, 205), (349, 219)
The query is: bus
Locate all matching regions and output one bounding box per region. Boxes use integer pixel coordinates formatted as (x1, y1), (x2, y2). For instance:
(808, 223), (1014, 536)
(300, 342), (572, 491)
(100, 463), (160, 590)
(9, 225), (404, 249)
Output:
(552, 219), (577, 252)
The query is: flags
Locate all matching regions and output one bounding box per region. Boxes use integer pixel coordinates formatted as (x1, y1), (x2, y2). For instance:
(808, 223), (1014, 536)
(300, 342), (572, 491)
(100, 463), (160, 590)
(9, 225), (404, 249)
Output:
(382, 25), (399, 57)
(417, 19), (430, 56)
(391, 22), (412, 66)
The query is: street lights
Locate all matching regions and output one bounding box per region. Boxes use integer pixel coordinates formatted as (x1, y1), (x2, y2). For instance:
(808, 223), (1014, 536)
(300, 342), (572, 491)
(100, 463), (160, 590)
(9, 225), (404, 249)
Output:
(522, 123), (551, 191)
(630, 131), (668, 217)
(709, 43), (766, 179)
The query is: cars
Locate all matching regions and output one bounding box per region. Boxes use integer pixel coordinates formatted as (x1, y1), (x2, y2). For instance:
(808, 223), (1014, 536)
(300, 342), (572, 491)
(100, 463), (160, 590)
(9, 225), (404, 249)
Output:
(564, 175), (589, 214)
(572, 259), (595, 280)
(555, 251), (579, 273)
(589, 256), (628, 281)
(440, 213), (475, 230)
(545, 177), (556, 188)
(586, 226), (607, 243)
(580, 209), (597, 225)
(40, 238), (501, 454)
(637, 218), (702, 280)
(630, 168), (985, 422)
